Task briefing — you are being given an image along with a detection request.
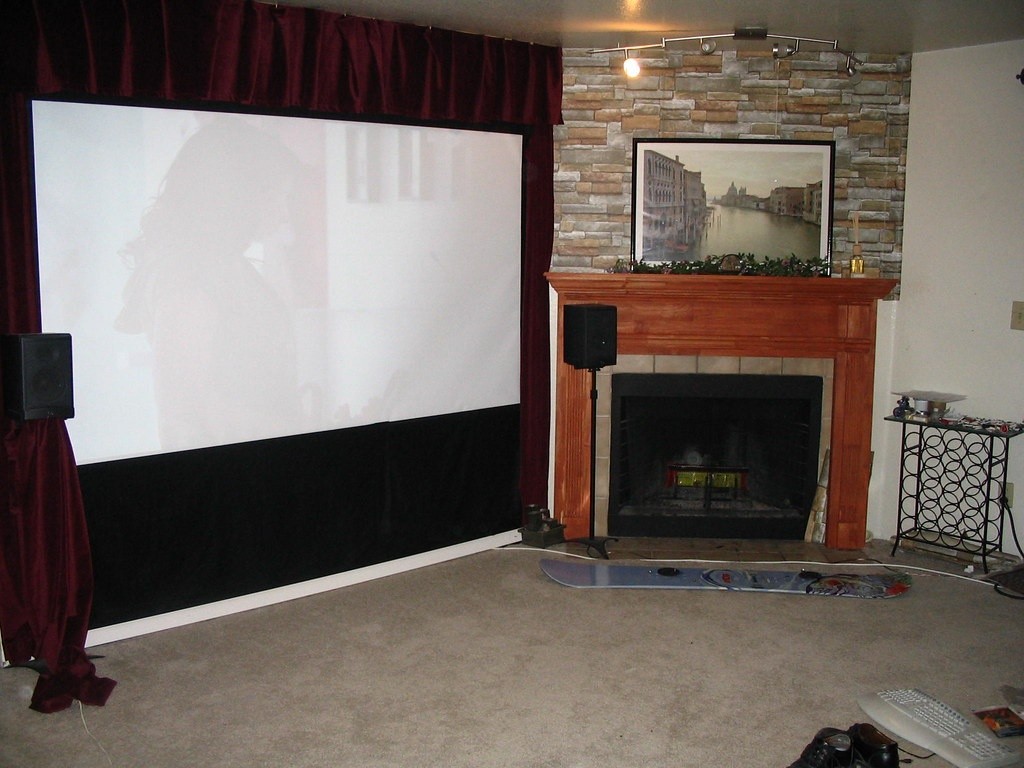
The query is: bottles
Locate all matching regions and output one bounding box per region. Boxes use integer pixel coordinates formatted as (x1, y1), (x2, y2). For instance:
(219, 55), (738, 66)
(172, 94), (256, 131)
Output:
(850, 245), (864, 274)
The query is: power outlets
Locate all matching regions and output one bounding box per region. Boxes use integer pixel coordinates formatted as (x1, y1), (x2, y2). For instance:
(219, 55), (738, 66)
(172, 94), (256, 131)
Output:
(998, 481), (1014, 508)
(1010, 301), (1024, 331)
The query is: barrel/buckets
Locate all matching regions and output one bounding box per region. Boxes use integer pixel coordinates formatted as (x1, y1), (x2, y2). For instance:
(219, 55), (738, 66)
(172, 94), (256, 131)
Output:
(667, 464), (710, 511)
(710, 467), (749, 510)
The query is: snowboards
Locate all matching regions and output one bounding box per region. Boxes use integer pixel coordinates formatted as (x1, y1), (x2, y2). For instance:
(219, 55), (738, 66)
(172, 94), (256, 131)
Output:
(538, 558), (914, 599)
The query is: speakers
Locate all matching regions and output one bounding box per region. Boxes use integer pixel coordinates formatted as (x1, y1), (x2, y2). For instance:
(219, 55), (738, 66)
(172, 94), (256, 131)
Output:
(2, 332), (75, 422)
(563, 304), (617, 370)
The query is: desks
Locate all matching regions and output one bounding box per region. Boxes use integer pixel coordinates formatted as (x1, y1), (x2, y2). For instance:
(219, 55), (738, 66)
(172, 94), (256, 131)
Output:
(884, 411), (1024, 574)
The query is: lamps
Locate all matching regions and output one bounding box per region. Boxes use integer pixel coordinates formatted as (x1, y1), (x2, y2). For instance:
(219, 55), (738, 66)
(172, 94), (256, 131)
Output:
(846, 56), (864, 86)
(699, 37), (716, 55)
(622, 49), (640, 78)
(773, 37), (800, 58)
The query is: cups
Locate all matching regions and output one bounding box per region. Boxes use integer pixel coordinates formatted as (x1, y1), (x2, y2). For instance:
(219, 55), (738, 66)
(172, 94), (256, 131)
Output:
(841, 261), (850, 278)
(832, 261), (841, 273)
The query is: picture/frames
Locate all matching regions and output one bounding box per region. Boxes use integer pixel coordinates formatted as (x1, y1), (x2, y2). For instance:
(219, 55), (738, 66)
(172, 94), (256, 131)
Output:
(630, 138), (836, 278)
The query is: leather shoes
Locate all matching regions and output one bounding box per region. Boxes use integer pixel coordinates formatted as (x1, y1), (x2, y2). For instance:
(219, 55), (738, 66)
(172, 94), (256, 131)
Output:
(847, 722), (900, 768)
(786, 728), (852, 768)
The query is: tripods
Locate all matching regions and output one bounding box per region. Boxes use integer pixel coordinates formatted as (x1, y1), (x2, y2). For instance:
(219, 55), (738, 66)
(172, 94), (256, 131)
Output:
(555, 367), (620, 562)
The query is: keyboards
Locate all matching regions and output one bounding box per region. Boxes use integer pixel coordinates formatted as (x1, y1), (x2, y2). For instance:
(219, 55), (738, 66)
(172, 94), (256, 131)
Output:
(857, 686), (1021, 768)
